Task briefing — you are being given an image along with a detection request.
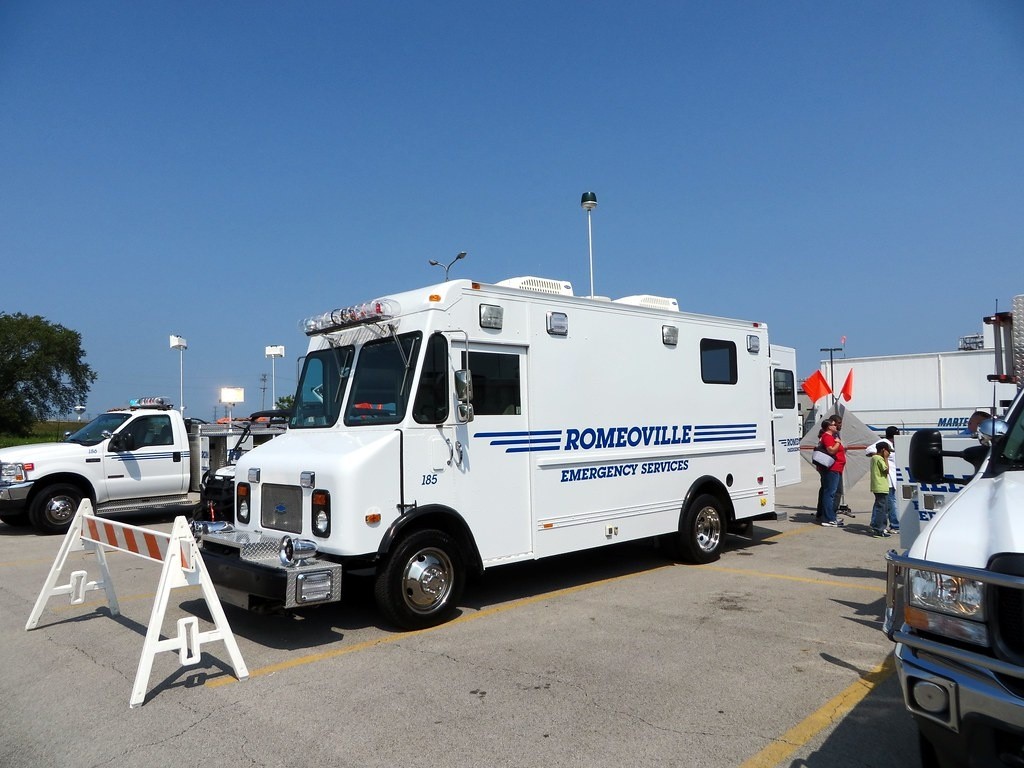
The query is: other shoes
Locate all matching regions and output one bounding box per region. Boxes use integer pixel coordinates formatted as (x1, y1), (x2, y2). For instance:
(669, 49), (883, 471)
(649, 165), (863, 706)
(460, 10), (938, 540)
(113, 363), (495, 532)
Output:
(872, 527), (889, 533)
(815, 518), (843, 524)
(890, 527), (901, 534)
(821, 519), (843, 527)
(873, 531), (891, 539)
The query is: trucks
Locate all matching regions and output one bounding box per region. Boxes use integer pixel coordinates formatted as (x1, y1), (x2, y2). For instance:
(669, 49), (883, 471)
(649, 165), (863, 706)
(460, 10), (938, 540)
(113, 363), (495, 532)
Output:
(883, 295), (1024, 768)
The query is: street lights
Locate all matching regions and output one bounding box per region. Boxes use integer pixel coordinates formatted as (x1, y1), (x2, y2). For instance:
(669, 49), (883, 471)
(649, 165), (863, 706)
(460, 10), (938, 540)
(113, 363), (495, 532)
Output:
(169, 334), (188, 418)
(428, 251), (467, 283)
(266, 345), (285, 409)
(820, 347), (842, 405)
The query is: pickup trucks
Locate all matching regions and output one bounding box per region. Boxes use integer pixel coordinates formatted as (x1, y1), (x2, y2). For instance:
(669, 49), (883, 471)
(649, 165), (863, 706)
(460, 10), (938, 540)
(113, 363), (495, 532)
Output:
(1, 396), (283, 535)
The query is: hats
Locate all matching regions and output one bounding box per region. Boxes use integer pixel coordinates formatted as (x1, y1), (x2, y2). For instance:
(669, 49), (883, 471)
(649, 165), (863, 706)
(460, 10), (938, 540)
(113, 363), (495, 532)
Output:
(876, 443), (895, 453)
(886, 426), (900, 437)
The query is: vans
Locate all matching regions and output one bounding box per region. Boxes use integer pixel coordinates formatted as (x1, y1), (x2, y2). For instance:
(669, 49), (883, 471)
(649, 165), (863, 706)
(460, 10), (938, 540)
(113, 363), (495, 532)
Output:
(184, 275), (803, 630)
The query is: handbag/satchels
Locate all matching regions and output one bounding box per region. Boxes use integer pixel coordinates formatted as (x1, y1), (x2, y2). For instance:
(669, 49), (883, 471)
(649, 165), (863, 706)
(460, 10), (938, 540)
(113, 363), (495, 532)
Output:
(812, 447), (836, 471)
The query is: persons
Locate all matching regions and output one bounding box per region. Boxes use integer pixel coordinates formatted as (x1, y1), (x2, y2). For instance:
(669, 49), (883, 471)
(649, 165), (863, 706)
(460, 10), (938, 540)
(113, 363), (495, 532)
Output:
(868, 442), (895, 539)
(816, 415), (846, 527)
(866, 426), (900, 533)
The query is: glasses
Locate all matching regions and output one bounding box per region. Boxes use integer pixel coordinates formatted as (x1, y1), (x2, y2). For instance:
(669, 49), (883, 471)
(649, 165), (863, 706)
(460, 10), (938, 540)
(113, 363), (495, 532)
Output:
(828, 424), (836, 429)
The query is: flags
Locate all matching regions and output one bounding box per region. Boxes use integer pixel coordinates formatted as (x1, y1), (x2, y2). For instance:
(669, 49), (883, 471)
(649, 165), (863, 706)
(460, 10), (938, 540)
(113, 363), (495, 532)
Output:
(800, 370), (832, 404)
(841, 368), (853, 402)
